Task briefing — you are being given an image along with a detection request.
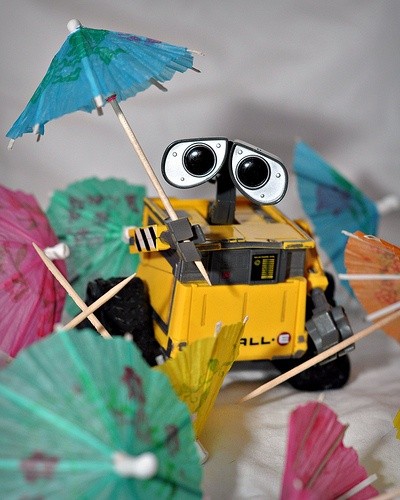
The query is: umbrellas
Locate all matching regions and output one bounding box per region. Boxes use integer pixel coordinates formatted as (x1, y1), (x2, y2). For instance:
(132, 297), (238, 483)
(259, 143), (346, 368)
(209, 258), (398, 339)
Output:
(0, 329), (207, 500)
(269, 402), (382, 500)
(238, 229), (400, 401)
(4, 18), (216, 291)
(29, 240), (250, 464)
(0, 183), (70, 362)
(286, 136), (398, 305)
(38, 175), (148, 317)
